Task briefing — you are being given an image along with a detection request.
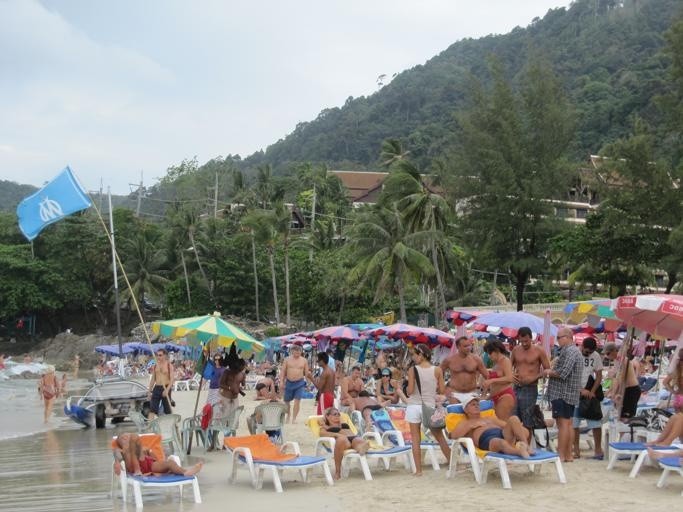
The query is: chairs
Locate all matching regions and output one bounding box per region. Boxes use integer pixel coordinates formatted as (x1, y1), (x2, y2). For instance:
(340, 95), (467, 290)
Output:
(224, 434), (333, 492)
(606, 423), (683, 488)
(247, 400), (287, 443)
(447, 394), (614, 489)
(128, 400), (244, 456)
(373, 411), (454, 471)
(112, 433), (202, 512)
(636, 376), (675, 416)
(175, 372), (201, 392)
(308, 414), (412, 482)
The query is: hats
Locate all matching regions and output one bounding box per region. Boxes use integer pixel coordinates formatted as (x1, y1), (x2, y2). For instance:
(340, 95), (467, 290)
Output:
(381, 368), (390, 374)
(601, 342), (617, 354)
(461, 397), (480, 410)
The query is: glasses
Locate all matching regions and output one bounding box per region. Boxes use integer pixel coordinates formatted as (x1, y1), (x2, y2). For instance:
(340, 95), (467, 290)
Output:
(329, 413), (340, 416)
(557, 335), (568, 339)
(382, 375), (389, 377)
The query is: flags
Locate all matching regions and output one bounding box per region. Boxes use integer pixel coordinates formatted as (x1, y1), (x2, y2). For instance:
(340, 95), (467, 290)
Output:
(14, 168), (91, 242)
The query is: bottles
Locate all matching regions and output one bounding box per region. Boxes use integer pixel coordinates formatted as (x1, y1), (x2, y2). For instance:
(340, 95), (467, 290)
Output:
(608, 411), (615, 425)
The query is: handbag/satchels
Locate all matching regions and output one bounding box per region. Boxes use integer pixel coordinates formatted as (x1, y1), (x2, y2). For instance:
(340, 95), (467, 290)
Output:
(522, 404), (546, 427)
(421, 402), (447, 429)
(580, 374), (604, 419)
(195, 351), (206, 375)
(644, 408), (673, 430)
(203, 358), (215, 380)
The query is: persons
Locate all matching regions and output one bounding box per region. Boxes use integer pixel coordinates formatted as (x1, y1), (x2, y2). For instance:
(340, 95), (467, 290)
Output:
(113, 433), (204, 478)
(576, 337), (603, 457)
(70, 354), (81, 379)
(97, 351), (152, 379)
(450, 396), (535, 458)
(203, 357), (246, 451)
(148, 348), (175, 421)
(482, 339), (515, 418)
(601, 334), (683, 462)
(442, 337), (486, 399)
(171, 352), (202, 380)
(38, 369), (68, 421)
(510, 327), (551, 444)
(544, 328), (583, 462)
(255, 344), (407, 481)
(406, 344), (451, 477)
(2, 353), (34, 369)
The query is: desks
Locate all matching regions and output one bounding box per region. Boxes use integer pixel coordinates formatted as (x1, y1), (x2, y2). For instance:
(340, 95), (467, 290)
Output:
(243, 375), (266, 390)
